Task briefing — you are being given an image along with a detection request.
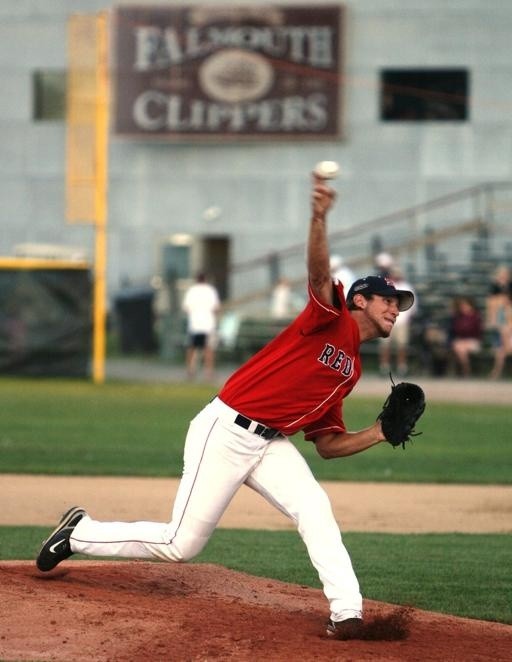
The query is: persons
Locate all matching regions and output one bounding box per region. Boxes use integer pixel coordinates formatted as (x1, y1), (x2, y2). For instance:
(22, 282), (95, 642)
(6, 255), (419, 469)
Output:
(329, 253), (512, 380)
(37, 173), (425, 636)
(182, 275), (222, 383)
(269, 276), (291, 317)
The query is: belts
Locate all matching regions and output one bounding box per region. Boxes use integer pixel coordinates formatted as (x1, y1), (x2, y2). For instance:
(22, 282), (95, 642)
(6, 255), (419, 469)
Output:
(209, 394), (285, 439)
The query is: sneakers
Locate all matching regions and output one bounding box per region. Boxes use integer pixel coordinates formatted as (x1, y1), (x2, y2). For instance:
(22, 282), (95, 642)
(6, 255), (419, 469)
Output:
(326, 618), (363, 636)
(36, 506), (88, 571)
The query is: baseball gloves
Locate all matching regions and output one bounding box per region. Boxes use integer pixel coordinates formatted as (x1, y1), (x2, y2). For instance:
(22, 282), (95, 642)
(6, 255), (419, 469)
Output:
(377, 372), (425, 450)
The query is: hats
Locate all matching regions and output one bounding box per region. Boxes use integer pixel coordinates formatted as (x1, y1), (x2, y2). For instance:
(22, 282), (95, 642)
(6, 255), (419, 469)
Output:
(345, 276), (414, 311)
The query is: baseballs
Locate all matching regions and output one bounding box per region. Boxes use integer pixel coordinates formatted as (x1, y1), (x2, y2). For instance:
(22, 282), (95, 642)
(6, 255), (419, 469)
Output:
(314, 161), (338, 178)
